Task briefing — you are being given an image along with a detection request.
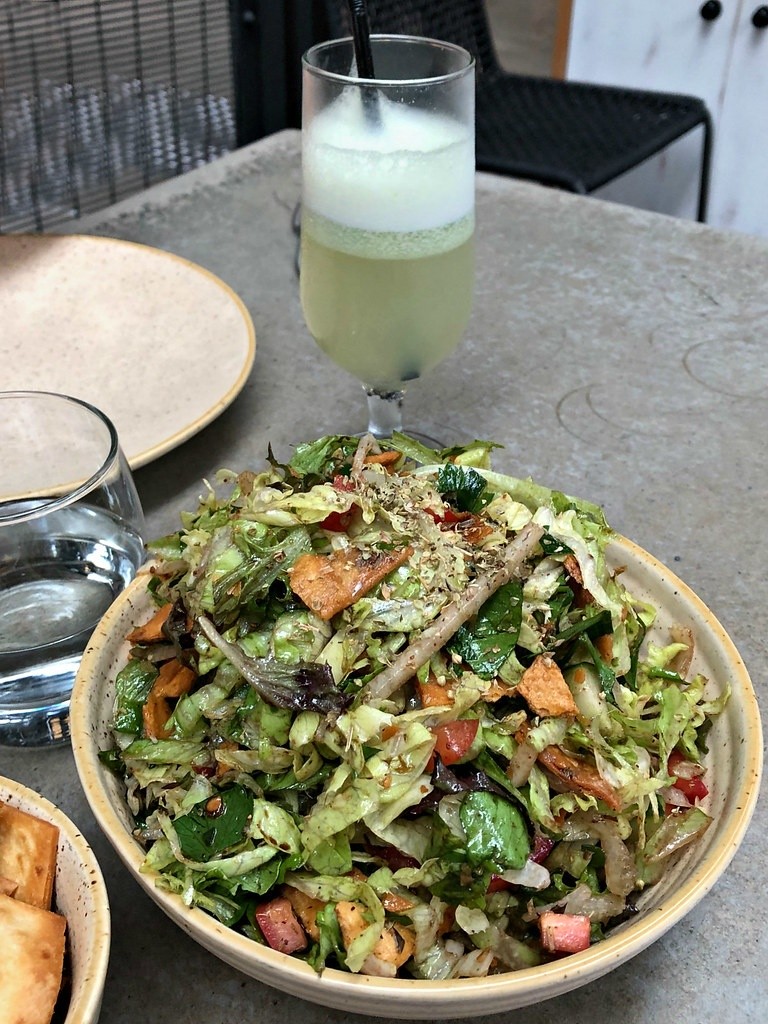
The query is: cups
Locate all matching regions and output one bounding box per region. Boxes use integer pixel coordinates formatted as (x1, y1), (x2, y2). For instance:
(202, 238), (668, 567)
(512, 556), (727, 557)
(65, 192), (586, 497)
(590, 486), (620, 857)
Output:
(0, 390), (147, 751)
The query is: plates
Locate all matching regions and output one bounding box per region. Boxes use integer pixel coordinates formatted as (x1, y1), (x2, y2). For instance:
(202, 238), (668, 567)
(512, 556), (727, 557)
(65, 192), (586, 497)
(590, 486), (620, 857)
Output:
(0, 234), (255, 502)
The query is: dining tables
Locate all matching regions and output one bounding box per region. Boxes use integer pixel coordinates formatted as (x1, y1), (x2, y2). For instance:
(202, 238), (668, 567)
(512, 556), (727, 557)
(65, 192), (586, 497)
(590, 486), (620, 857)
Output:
(0, 128), (767, 1024)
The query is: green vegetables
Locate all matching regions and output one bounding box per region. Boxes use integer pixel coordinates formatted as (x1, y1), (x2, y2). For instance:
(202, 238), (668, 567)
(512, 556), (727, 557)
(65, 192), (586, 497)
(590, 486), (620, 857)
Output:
(110, 424), (732, 974)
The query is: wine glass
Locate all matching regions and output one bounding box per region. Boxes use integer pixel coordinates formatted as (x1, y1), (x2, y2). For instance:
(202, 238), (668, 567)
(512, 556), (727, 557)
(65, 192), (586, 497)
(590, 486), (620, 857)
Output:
(299, 37), (476, 471)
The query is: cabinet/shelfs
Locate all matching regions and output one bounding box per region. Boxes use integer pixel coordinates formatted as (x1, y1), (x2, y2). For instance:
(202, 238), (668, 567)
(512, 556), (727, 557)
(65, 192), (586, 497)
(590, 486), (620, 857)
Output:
(562, 0), (765, 235)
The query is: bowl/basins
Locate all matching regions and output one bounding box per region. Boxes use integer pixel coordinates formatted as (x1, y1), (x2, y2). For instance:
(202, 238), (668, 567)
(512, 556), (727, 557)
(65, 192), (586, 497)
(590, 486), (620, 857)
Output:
(67, 468), (763, 1020)
(0, 774), (110, 1024)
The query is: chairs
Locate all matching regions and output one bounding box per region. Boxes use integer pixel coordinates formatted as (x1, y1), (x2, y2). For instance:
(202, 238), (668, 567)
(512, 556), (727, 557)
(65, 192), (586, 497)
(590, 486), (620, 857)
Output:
(305, 0), (714, 225)
